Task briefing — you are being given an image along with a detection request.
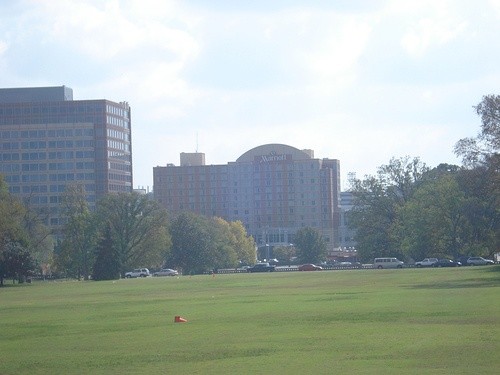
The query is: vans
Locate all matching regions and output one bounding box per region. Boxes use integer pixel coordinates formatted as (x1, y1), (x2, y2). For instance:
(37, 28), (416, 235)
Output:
(415, 258), (440, 268)
(374, 257), (403, 270)
(467, 256), (495, 266)
(125, 267), (150, 279)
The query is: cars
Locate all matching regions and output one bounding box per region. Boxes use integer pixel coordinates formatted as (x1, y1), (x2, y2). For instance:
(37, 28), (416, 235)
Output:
(152, 268), (179, 278)
(298, 264), (323, 271)
(438, 260), (464, 268)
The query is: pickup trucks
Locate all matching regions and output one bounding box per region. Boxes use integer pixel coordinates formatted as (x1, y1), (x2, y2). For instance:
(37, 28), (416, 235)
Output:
(246, 263), (277, 275)
